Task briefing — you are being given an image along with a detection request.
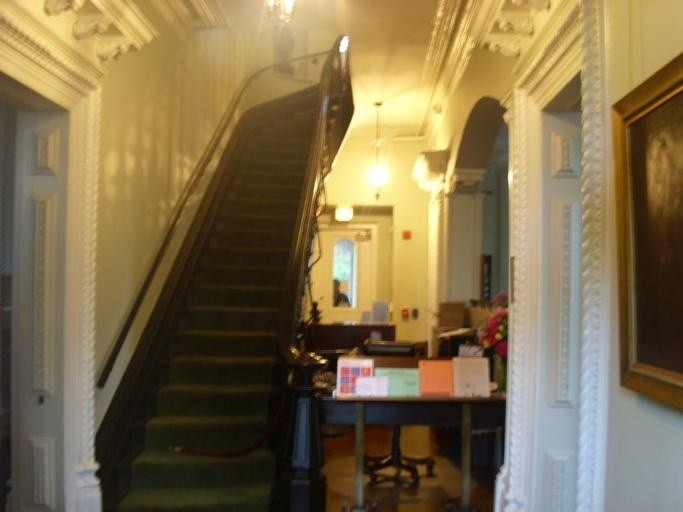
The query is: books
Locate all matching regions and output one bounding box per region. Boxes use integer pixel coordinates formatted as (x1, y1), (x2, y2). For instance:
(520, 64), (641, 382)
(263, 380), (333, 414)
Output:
(417, 357), (456, 399)
(335, 356), (375, 398)
(450, 354), (492, 399)
(373, 365), (421, 398)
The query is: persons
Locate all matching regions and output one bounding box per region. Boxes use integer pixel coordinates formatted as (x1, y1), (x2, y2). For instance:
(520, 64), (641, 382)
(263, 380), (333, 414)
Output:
(332, 278), (351, 307)
(478, 291), (508, 391)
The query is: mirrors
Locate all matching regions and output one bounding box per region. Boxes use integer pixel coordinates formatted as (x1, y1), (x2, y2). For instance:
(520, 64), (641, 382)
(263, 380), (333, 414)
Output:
(330, 237), (356, 308)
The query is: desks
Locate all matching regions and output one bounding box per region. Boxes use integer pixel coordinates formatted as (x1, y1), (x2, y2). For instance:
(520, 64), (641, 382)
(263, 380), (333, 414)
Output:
(323, 354), (505, 512)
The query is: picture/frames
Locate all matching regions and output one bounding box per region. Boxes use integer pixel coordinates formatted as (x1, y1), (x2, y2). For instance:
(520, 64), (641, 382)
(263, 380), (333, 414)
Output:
(609, 50), (683, 412)
